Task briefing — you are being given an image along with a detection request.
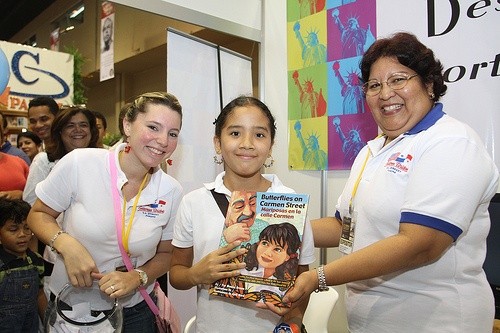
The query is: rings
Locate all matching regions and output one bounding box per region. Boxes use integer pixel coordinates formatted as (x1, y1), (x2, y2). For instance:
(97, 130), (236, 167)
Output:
(110, 286), (115, 292)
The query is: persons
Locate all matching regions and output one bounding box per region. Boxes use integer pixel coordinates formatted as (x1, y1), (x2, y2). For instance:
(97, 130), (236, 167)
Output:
(0, 199), (48, 333)
(94, 103), (132, 150)
(24, 107), (99, 298)
(0, 112), (30, 200)
(29, 97), (60, 150)
(103, 18), (113, 51)
(169, 97), (316, 332)
(26, 93), (183, 333)
(17, 131), (42, 162)
(256, 32), (500, 333)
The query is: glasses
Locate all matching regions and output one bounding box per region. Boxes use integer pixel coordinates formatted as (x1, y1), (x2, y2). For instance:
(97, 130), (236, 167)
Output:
(361, 71), (419, 98)
(60, 103), (87, 112)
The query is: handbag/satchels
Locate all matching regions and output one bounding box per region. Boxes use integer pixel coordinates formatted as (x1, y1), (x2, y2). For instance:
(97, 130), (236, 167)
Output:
(154, 281), (182, 333)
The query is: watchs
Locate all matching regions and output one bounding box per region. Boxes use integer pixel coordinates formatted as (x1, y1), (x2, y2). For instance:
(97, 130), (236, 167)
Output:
(135, 269), (148, 289)
(317, 265), (329, 292)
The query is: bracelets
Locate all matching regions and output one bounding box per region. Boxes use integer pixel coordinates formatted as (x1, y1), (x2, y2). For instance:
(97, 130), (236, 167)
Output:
(51, 231), (68, 254)
